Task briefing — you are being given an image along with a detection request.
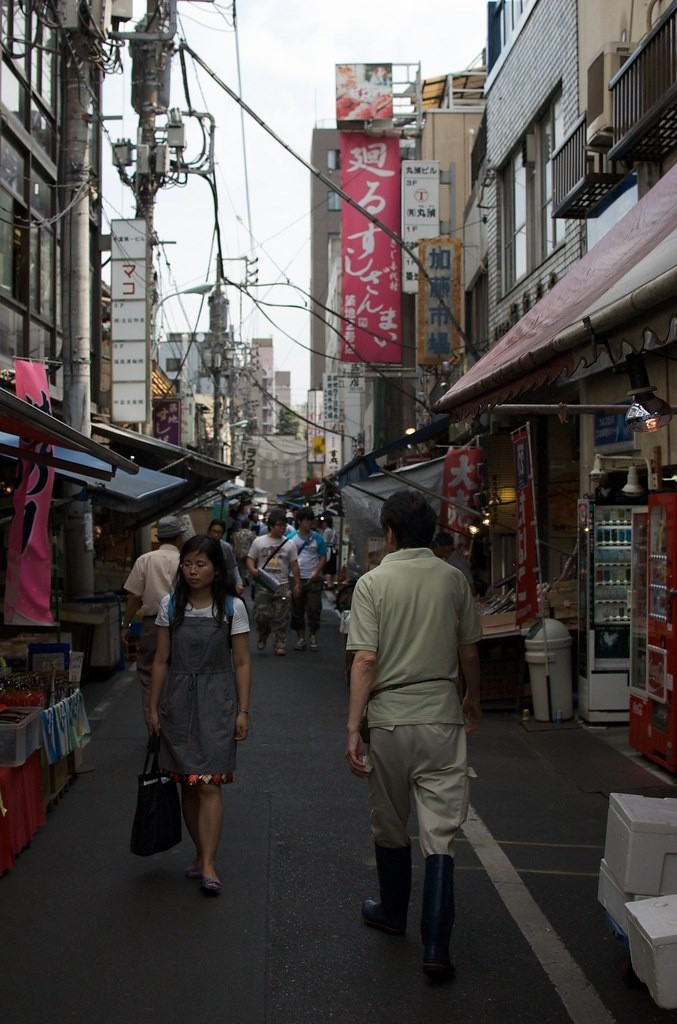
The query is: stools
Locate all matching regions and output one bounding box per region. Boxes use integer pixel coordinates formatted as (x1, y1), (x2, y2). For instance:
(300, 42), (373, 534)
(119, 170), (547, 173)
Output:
(125, 637), (141, 661)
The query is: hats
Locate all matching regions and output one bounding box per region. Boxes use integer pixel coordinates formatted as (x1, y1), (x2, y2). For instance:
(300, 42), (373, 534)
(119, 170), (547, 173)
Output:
(155, 516), (186, 537)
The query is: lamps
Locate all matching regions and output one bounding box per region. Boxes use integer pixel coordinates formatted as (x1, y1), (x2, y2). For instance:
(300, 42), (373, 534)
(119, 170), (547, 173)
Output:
(622, 465), (646, 497)
(623, 356), (673, 434)
(589, 452), (609, 483)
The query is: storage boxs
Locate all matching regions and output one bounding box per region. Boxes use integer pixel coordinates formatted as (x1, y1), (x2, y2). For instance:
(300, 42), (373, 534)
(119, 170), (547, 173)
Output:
(624, 894), (677, 1010)
(0, 707), (44, 768)
(480, 611), (520, 637)
(597, 858), (659, 936)
(550, 579), (578, 620)
(604, 793), (677, 896)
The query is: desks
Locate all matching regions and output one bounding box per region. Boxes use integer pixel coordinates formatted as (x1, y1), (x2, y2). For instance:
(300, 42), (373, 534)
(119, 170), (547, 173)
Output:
(0, 749), (46, 876)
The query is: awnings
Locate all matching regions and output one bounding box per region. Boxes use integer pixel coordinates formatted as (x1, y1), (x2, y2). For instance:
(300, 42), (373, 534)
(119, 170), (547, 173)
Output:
(1, 386), (243, 531)
(432, 162), (677, 423)
(277, 416), (451, 505)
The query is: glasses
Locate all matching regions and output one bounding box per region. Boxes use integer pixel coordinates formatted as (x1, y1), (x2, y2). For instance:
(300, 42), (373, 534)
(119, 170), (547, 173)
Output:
(275, 524), (287, 527)
(180, 563), (209, 569)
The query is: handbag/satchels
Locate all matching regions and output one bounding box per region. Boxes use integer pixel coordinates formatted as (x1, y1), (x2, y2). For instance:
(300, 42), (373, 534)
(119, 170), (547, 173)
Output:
(129, 732), (182, 857)
(250, 584), (255, 600)
(359, 706), (370, 743)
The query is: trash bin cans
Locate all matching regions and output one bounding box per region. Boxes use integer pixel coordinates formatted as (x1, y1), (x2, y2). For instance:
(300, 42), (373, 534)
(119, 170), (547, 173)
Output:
(523, 617), (573, 724)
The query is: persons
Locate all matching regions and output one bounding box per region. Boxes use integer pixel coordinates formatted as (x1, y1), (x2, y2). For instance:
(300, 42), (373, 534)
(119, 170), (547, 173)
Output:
(121, 516), (188, 752)
(207, 506), (338, 656)
(147, 534), (251, 891)
(345, 490), (483, 981)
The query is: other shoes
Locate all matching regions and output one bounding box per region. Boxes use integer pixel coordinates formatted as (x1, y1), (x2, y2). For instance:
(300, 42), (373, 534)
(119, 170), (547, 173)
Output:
(294, 639), (307, 650)
(310, 644), (319, 650)
(201, 877), (222, 891)
(274, 643), (285, 654)
(257, 640), (264, 650)
(184, 865), (201, 878)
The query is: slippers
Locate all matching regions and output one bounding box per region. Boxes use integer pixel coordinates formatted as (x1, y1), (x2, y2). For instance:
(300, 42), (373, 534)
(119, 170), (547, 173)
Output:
(420, 854), (455, 978)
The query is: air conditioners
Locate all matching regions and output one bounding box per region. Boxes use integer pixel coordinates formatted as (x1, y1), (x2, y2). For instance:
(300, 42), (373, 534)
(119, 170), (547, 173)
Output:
(583, 41), (640, 147)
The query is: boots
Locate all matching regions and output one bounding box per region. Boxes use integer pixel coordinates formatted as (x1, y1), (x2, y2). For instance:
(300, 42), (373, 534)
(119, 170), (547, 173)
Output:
(361, 837), (411, 934)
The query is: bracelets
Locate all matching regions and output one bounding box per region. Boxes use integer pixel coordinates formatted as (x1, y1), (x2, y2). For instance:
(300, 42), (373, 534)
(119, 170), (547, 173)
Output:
(239, 709), (250, 714)
(122, 621), (131, 628)
(295, 583), (302, 588)
